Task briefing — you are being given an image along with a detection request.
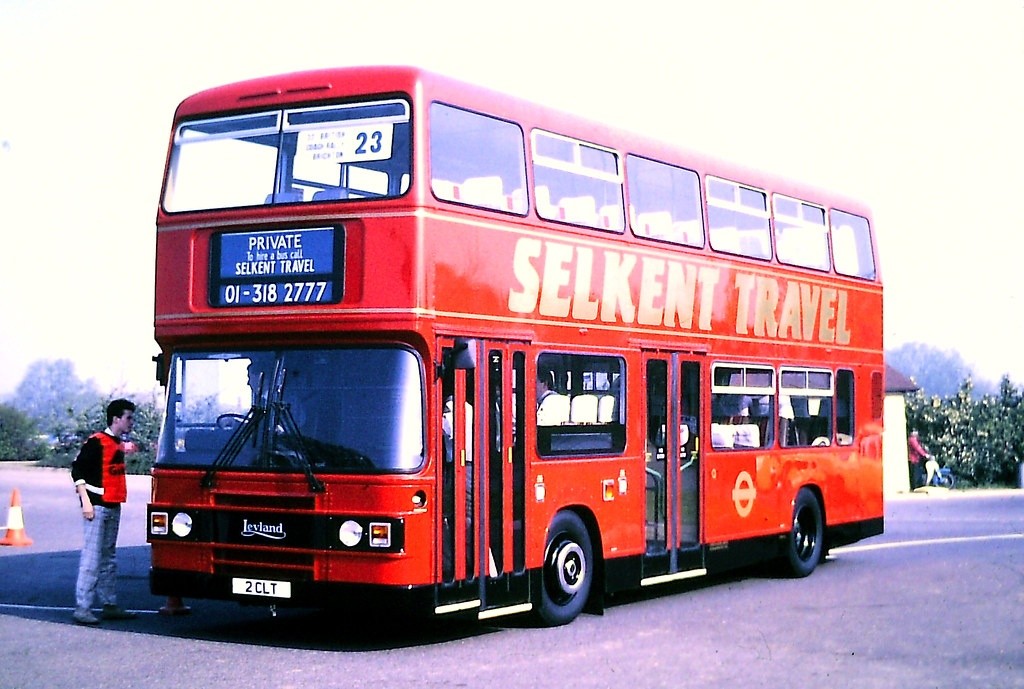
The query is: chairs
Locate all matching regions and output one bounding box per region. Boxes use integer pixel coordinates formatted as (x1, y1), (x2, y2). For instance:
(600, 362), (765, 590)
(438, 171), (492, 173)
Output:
(537, 395), (853, 448)
(265, 174), (861, 278)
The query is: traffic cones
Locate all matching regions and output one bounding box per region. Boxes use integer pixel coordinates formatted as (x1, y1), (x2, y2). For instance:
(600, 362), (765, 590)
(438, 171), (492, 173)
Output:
(158, 596), (190, 617)
(0, 489), (36, 547)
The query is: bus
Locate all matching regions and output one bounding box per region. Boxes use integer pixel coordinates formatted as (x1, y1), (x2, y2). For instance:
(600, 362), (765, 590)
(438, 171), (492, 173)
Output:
(145, 62), (888, 629)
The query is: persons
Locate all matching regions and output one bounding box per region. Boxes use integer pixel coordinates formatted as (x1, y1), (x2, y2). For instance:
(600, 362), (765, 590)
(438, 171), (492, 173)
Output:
(441, 367), (497, 581)
(535, 363), (570, 426)
(219, 360), (307, 435)
(907, 427), (930, 490)
(70, 399), (136, 624)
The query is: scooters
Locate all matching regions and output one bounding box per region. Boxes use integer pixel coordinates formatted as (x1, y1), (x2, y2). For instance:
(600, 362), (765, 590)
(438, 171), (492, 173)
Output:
(921, 456), (955, 489)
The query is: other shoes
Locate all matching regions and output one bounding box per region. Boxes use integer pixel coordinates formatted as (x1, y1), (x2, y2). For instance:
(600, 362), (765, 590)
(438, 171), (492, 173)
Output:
(73, 611), (98, 624)
(103, 608), (136, 619)
